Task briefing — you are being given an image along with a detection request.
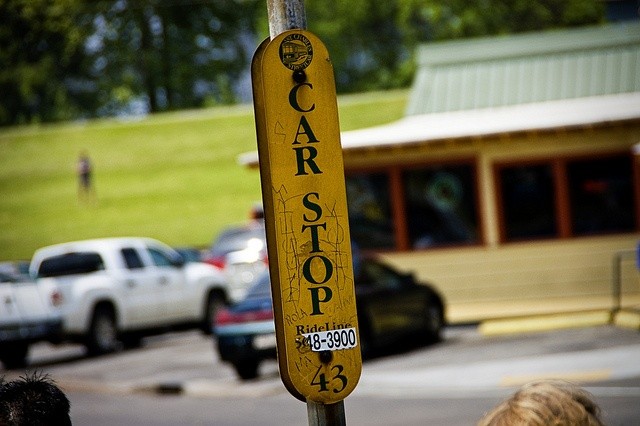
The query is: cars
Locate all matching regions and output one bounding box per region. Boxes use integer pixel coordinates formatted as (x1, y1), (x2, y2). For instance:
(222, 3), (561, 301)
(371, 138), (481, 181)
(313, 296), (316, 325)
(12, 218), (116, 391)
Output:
(214, 259), (443, 379)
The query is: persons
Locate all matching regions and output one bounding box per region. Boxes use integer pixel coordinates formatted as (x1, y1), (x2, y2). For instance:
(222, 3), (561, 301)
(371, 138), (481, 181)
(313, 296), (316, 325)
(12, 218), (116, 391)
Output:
(478, 378), (604, 426)
(0, 371), (74, 426)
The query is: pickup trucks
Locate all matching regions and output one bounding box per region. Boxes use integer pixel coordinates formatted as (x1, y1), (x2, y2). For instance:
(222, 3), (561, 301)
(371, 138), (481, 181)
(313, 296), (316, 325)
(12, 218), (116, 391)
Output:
(0, 237), (224, 370)
